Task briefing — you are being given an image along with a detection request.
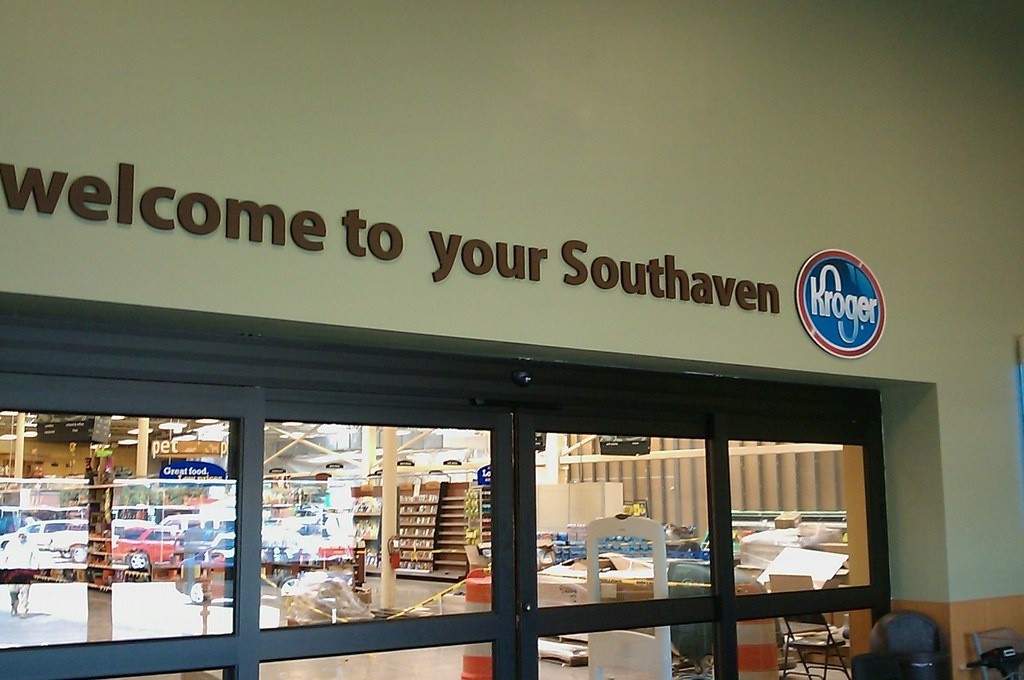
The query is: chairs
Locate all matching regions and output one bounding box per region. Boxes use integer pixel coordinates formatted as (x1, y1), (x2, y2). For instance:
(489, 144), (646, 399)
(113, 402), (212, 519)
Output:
(782, 612), (851, 680)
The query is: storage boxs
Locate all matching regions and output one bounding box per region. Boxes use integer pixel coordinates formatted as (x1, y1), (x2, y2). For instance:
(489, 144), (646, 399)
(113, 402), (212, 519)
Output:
(352, 586), (371, 605)
(774, 512), (802, 529)
(466, 523), (669, 641)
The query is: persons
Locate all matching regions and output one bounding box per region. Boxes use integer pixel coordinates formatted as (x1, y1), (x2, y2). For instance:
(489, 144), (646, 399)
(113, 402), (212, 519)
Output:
(0, 530), (41, 618)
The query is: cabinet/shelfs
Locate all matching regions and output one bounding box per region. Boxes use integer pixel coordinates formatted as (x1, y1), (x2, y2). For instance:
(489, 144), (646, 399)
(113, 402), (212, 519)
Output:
(260, 473), (491, 584)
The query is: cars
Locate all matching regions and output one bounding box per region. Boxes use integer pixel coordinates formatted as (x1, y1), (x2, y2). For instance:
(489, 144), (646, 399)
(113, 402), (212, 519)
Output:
(1, 503), (362, 609)
